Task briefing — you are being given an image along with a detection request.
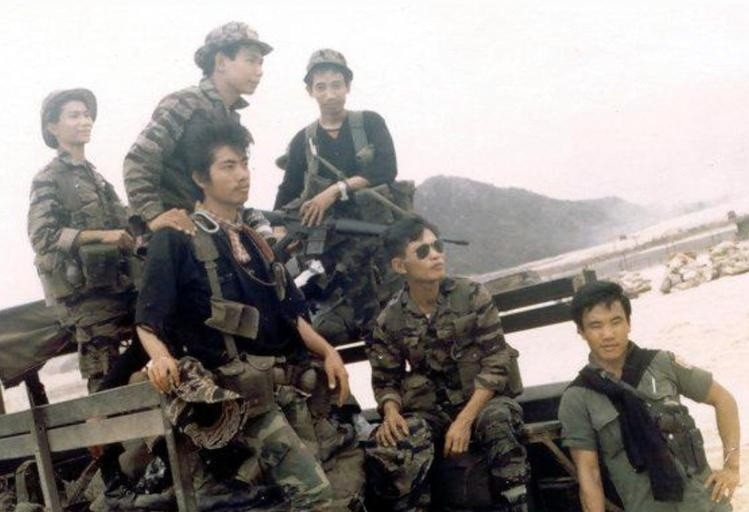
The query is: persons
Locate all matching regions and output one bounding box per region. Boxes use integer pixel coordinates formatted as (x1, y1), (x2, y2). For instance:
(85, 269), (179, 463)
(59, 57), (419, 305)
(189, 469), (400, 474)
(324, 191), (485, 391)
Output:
(363, 214), (536, 509)
(135, 117), (371, 508)
(268, 48), (408, 345)
(728, 210), (749, 241)
(557, 280), (740, 511)
(124, 22), (272, 238)
(30, 85), (171, 506)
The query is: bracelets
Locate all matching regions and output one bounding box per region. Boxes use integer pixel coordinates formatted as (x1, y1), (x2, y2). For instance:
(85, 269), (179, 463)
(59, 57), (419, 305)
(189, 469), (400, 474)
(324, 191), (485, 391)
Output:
(333, 180), (350, 203)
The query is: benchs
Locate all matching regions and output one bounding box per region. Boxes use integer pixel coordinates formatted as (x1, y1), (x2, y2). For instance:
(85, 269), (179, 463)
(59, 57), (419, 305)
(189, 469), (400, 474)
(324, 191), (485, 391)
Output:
(464, 421), (626, 512)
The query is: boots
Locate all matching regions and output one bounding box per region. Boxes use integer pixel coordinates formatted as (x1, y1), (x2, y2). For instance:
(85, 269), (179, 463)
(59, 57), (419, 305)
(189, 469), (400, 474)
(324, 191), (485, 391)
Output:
(102, 471), (174, 511)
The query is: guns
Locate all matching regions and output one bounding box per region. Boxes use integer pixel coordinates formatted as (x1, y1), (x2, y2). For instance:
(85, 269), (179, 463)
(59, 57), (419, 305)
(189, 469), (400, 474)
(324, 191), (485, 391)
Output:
(242, 207), (469, 260)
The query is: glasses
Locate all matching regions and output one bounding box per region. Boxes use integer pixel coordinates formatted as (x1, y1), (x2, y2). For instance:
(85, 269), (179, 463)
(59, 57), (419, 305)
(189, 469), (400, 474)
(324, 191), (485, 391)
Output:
(402, 240), (444, 268)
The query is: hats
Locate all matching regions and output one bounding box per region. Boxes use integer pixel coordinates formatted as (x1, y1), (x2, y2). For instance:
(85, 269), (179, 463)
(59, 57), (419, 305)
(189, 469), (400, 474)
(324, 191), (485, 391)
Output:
(163, 356), (247, 450)
(41, 89), (97, 149)
(195, 24), (273, 68)
(304, 51), (351, 82)
